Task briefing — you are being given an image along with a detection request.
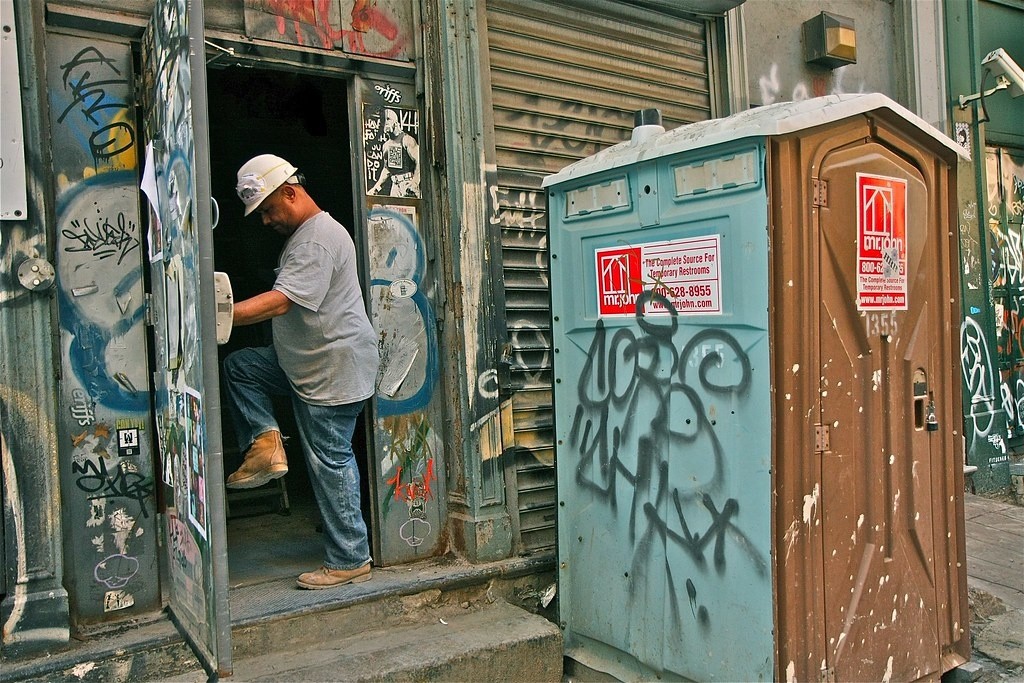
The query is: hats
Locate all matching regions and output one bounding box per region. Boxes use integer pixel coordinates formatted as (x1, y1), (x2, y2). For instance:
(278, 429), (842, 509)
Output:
(235, 153), (297, 218)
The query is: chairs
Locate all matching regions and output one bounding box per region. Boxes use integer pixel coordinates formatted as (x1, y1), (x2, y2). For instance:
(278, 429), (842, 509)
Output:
(220, 403), (291, 519)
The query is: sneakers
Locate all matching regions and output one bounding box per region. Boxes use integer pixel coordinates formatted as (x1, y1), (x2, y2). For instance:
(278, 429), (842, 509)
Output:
(226, 431), (288, 488)
(296, 560), (372, 589)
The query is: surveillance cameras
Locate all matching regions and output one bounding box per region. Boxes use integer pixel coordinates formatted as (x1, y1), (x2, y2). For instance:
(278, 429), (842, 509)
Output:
(981, 48), (1024, 99)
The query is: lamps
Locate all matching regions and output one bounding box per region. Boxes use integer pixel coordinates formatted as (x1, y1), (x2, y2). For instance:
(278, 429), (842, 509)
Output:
(803, 10), (856, 71)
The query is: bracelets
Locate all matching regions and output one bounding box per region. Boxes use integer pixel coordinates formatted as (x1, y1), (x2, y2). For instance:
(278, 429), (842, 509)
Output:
(374, 184), (382, 192)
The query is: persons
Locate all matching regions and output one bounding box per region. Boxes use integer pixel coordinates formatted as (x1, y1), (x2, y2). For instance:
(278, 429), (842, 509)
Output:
(224, 153), (380, 589)
(368, 109), (421, 197)
